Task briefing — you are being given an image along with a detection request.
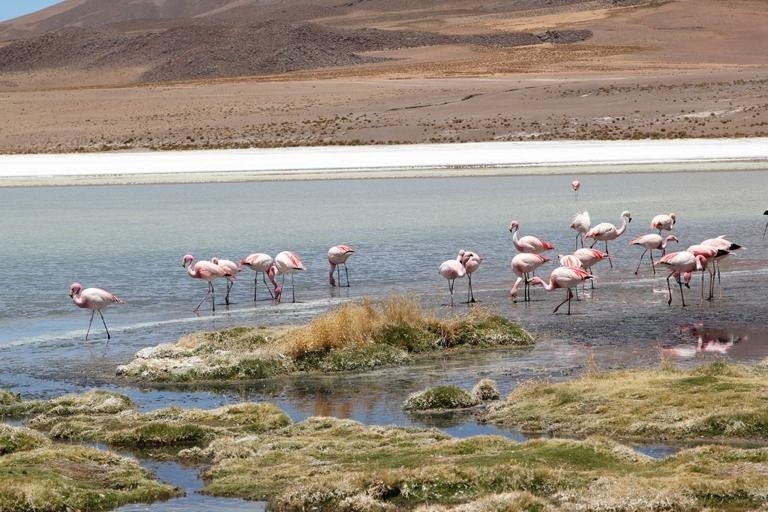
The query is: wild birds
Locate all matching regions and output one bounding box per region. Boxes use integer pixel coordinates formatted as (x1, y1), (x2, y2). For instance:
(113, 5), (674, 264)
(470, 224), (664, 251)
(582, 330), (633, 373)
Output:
(211, 257), (243, 305)
(509, 210), (632, 315)
(628, 213), (747, 307)
(327, 245), (355, 287)
(181, 255), (233, 312)
(438, 249), (484, 306)
(240, 253), (281, 302)
(69, 283), (125, 339)
(572, 181), (581, 200)
(268, 250), (307, 303)
